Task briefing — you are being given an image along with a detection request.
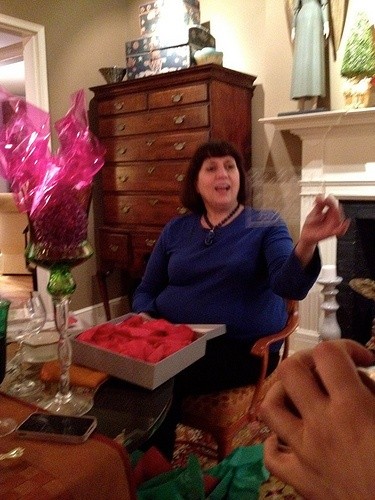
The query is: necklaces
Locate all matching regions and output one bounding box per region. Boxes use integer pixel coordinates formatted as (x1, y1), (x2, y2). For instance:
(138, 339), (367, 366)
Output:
(203, 202), (240, 246)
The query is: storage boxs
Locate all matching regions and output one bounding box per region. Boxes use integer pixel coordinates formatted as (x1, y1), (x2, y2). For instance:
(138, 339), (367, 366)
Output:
(69, 313), (226, 389)
(125, 0), (215, 79)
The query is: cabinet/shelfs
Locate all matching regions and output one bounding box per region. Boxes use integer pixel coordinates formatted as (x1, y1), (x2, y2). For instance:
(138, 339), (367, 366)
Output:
(89, 63), (257, 322)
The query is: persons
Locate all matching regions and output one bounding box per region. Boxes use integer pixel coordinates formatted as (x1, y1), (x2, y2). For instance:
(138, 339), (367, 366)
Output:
(132, 142), (350, 464)
(291, 0), (331, 111)
(259, 339), (375, 500)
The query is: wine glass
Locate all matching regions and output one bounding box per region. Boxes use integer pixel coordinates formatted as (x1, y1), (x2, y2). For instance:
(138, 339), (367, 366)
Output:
(0, 298), (18, 437)
(22, 182), (94, 418)
(0, 290), (48, 396)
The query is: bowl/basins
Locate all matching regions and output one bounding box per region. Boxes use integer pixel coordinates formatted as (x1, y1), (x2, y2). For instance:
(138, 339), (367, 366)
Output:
(99, 67), (126, 83)
(195, 52), (223, 65)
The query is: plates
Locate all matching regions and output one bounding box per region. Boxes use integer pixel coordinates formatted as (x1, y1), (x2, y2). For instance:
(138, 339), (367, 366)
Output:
(24, 353), (58, 363)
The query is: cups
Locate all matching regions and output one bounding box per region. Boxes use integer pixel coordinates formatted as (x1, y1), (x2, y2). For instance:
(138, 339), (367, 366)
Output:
(23, 331), (60, 359)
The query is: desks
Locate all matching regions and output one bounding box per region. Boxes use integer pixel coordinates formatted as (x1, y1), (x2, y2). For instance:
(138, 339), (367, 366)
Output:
(0, 327), (173, 499)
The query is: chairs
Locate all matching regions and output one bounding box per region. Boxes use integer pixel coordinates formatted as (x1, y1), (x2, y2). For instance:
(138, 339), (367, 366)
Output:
(94, 251), (297, 463)
